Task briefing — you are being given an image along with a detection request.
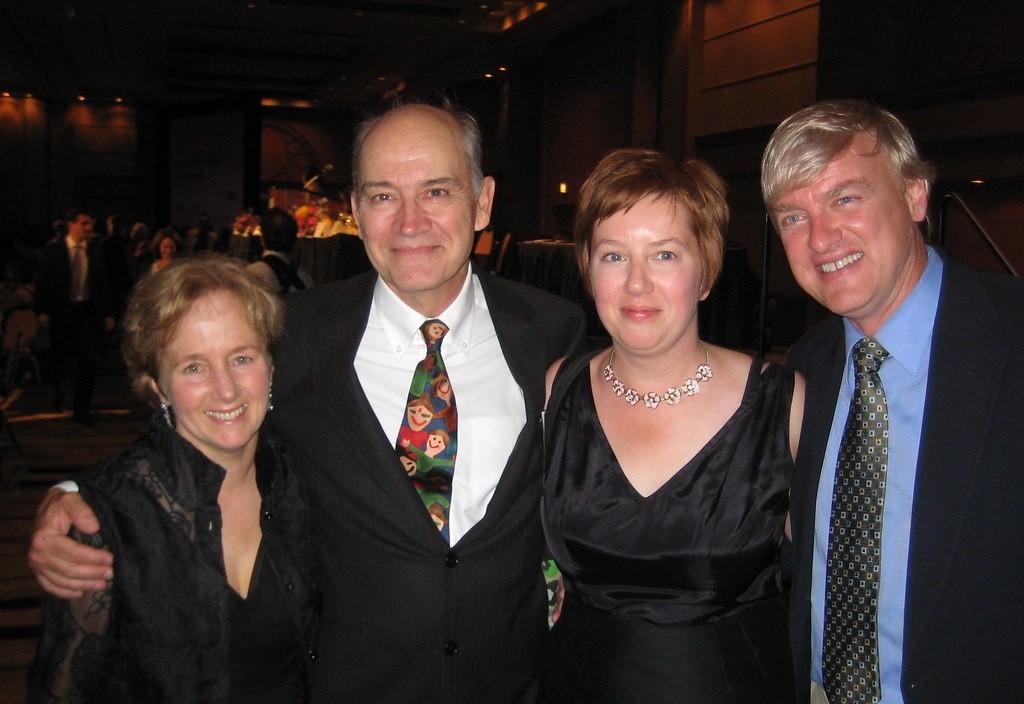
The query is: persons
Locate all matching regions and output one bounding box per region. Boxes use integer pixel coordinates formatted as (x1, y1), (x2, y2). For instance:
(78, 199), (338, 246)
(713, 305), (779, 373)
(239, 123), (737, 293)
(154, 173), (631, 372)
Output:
(32, 208), (114, 426)
(760, 100), (1024, 703)
(12, 211), (219, 283)
(24, 250), (320, 703)
(250, 207), (345, 296)
(537, 150), (806, 704)
(31, 102), (588, 704)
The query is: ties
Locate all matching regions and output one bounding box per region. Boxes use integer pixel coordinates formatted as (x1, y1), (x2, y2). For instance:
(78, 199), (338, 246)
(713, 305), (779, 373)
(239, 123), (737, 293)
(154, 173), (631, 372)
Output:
(821, 336), (889, 704)
(70, 244), (86, 298)
(394, 318), (459, 549)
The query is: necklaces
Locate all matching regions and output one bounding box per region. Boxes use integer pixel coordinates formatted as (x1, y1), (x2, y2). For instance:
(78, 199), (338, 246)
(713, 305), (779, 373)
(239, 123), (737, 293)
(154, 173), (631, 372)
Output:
(603, 339), (713, 408)
